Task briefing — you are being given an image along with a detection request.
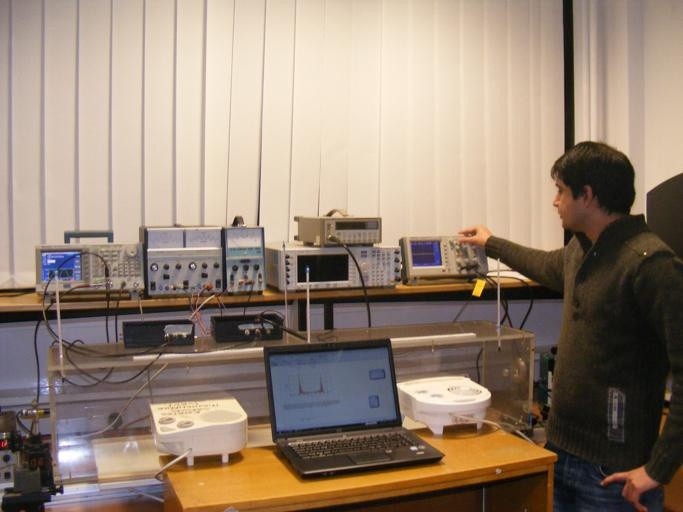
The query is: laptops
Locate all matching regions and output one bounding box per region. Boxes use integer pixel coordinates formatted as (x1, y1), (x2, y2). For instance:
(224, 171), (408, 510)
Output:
(263, 338), (446, 478)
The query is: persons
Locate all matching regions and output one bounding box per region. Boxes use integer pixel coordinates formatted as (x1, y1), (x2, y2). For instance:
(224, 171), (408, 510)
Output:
(457, 141), (683, 512)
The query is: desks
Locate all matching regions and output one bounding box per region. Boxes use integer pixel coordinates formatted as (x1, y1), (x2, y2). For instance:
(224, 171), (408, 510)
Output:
(156, 418), (557, 508)
